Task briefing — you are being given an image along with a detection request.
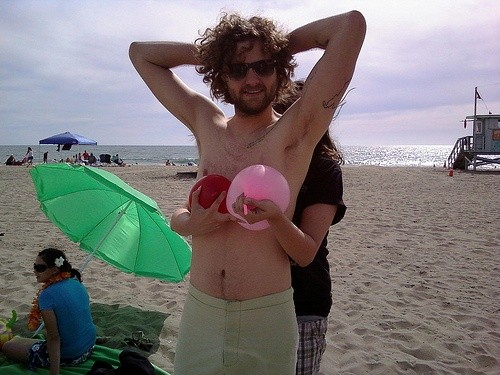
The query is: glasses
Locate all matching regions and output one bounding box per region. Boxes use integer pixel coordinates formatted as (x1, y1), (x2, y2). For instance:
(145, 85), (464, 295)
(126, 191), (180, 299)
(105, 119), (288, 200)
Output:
(219, 59), (279, 81)
(34, 263), (52, 272)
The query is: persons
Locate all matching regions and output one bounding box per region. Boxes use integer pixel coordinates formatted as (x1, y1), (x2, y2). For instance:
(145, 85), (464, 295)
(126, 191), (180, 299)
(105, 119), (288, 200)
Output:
(5, 146), (125, 166)
(0, 248), (97, 375)
(170, 80), (348, 375)
(129, 10), (366, 375)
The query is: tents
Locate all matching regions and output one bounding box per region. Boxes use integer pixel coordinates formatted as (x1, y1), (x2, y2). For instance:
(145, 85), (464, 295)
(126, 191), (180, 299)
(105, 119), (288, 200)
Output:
(37, 131), (98, 164)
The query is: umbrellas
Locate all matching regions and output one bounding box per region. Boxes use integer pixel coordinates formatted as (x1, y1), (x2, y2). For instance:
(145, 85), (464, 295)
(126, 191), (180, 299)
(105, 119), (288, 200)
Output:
(29, 160), (191, 335)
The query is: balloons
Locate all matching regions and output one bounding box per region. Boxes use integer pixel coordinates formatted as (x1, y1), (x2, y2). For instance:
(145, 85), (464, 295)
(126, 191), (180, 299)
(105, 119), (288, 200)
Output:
(189, 175), (232, 215)
(227, 164), (290, 231)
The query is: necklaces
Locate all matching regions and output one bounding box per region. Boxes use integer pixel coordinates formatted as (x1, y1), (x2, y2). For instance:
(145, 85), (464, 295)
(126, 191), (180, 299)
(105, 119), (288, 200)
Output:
(27, 271), (71, 330)
